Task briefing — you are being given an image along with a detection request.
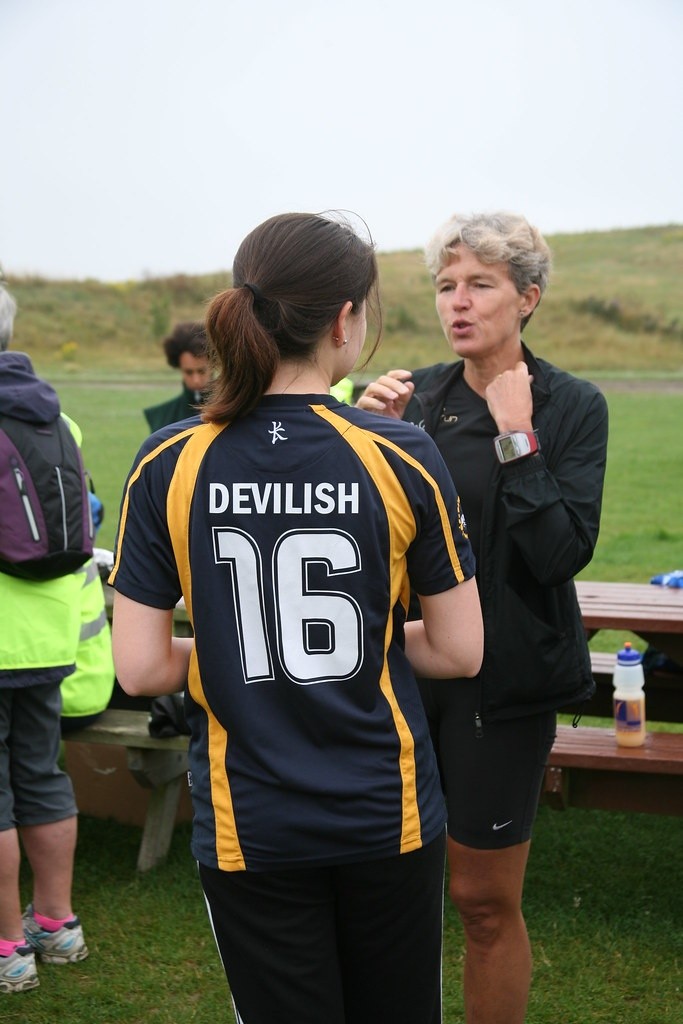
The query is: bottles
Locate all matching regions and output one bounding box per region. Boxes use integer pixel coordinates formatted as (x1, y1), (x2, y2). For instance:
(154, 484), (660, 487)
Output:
(613, 642), (645, 748)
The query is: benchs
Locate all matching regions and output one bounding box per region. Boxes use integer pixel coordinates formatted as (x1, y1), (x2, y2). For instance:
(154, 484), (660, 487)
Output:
(101, 578), (683, 700)
(56, 723), (683, 878)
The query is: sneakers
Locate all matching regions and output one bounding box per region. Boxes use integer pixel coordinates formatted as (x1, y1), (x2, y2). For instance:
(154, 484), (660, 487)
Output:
(23, 902), (89, 964)
(1, 943), (39, 994)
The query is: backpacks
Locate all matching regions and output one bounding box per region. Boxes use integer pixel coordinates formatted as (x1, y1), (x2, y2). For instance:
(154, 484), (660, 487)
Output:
(1, 414), (93, 581)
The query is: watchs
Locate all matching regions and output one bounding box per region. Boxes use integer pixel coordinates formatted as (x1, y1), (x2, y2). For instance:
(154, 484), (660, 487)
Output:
(493, 431), (543, 466)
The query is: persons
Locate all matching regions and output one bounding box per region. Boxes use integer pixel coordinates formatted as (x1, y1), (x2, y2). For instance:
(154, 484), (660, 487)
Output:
(109, 211), (486, 1024)
(145, 323), (223, 435)
(52, 410), (119, 736)
(355, 211), (611, 1024)
(3, 285), (99, 997)
